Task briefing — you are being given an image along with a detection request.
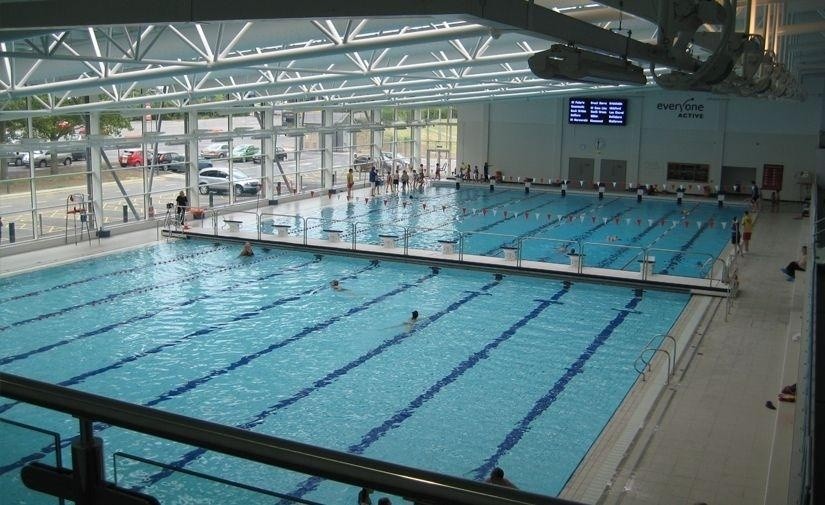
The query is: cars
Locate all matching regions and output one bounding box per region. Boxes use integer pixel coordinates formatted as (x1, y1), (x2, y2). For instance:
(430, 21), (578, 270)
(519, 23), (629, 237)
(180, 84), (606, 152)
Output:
(353, 149), (412, 173)
(8, 124), (287, 199)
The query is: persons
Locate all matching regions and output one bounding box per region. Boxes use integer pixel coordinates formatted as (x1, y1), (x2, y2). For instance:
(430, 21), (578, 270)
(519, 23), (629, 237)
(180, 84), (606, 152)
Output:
(403, 309), (421, 333)
(481, 466), (519, 489)
(565, 248), (586, 258)
(175, 189), (190, 227)
(780, 246), (808, 282)
(240, 240), (255, 257)
(346, 168), (355, 197)
(751, 181), (760, 210)
(771, 191), (776, 204)
(741, 211), (753, 252)
(358, 485), (374, 505)
(367, 160), (497, 197)
(731, 216), (744, 257)
(328, 279), (351, 294)
(776, 188), (780, 202)
